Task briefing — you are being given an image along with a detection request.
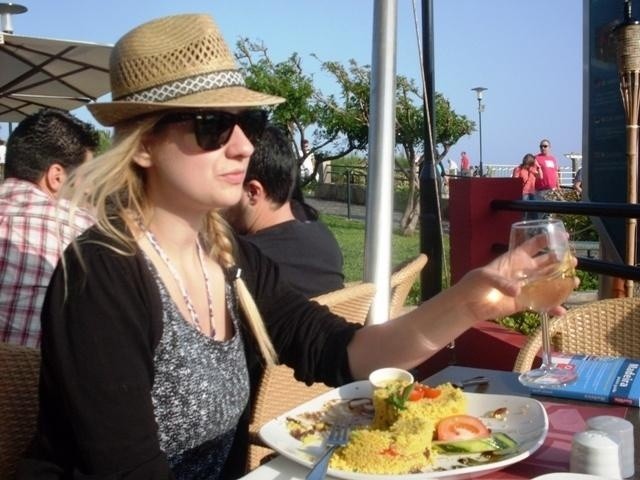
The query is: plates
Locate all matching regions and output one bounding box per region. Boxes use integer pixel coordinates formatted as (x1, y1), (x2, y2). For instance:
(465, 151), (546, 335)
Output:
(257, 379), (551, 480)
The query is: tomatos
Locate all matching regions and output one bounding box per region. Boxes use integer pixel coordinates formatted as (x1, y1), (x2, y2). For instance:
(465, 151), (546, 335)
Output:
(436, 415), (490, 443)
(404, 380), (440, 400)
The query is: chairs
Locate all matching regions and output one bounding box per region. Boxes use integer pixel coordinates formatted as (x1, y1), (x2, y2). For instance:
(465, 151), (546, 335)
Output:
(0, 344), (52, 480)
(248, 282), (375, 469)
(342, 253), (427, 321)
(512, 297), (640, 375)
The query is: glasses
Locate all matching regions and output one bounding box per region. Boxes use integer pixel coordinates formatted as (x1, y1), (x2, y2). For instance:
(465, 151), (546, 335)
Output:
(540, 145), (547, 148)
(158, 108), (268, 151)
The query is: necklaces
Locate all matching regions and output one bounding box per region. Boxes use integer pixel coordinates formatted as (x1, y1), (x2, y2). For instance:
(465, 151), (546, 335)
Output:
(129, 209), (217, 342)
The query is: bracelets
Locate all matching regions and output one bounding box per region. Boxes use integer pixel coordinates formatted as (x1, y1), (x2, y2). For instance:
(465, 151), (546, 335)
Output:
(405, 311), (455, 353)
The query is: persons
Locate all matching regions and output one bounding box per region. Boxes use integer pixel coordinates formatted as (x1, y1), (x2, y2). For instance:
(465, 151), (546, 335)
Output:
(0, 141), (7, 178)
(534, 140), (560, 220)
(15, 13), (582, 480)
(435, 152), (469, 196)
(574, 168), (582, 194)
(0, 109), (98, 352)
(295, 139), (314, 181)
(229, 124), (346, 297)
(513, 154), (543, 222)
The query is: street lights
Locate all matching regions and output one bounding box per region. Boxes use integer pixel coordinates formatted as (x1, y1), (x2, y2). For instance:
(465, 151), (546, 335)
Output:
(563, 152), (583, 183)
(470, 87), (489, 176)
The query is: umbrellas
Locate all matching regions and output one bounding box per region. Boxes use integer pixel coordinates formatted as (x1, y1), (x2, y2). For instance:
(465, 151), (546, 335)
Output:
(0, 2), (114, 135)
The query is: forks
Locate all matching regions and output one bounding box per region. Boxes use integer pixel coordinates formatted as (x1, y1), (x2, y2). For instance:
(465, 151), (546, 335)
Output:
(306, 414), (349, 480)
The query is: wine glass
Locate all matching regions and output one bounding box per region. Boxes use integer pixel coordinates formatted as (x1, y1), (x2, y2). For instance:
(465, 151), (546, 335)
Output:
(507, 220), (578, 387)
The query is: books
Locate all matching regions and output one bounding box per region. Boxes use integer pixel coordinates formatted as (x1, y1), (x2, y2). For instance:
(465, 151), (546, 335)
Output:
(529, 352), (640, 407)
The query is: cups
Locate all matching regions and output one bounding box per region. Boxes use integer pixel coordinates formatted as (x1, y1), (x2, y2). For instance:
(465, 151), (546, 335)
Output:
(370, 368), (415, 389)
(569, 429), (621, 480)
(532, 471), (612, 480)
(584, 415), (635, 480)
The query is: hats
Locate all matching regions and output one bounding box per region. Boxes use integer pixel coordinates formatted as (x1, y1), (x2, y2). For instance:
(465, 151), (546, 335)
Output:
(85, 13), (288, 125)
